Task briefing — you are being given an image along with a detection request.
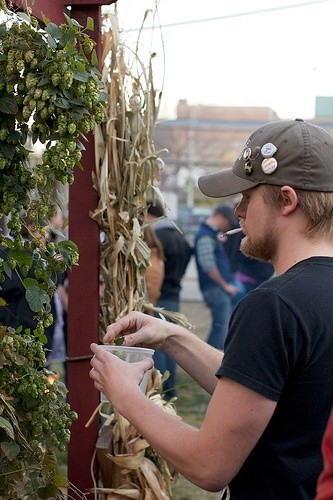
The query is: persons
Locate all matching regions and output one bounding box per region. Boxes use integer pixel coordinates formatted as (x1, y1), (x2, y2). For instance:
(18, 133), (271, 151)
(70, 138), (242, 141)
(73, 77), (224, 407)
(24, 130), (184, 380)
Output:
(99, 118), (333, 500)
(0, 198), (333, 451)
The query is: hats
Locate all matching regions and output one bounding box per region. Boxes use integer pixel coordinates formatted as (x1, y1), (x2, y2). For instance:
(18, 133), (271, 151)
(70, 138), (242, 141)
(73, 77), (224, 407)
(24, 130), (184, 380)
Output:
(197, 118), (333, 199)
(215, 203), (236, 227)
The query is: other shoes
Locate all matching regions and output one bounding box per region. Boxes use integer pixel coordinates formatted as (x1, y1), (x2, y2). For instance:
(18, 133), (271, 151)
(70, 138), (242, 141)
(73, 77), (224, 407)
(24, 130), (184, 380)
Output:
(163, 391), (177, 404)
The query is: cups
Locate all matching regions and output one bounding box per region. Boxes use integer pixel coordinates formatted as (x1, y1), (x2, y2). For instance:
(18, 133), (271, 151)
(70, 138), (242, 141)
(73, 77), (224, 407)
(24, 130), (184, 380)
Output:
(98, 345), (155, 402)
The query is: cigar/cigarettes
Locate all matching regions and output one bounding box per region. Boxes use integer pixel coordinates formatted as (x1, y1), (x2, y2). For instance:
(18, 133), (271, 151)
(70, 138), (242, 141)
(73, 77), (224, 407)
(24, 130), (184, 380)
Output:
(225, 227), (242, 236)
